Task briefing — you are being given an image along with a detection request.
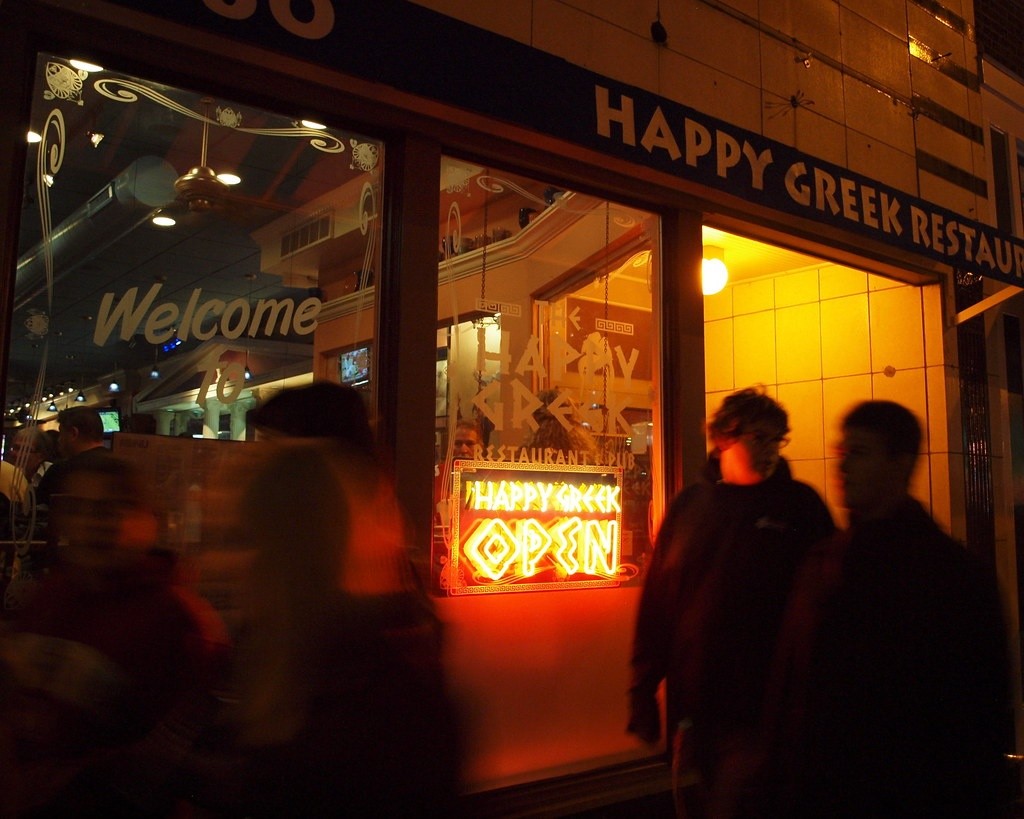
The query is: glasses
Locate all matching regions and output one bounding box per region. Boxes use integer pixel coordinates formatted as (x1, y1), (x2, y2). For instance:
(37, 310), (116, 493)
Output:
(728, 428), (793, 450)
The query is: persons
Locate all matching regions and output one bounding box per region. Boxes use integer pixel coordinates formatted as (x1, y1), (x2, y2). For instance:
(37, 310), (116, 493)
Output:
(784, 398), (1024, 819)
(2, 368), (475, 816)
(625, 386), (845, 819)
